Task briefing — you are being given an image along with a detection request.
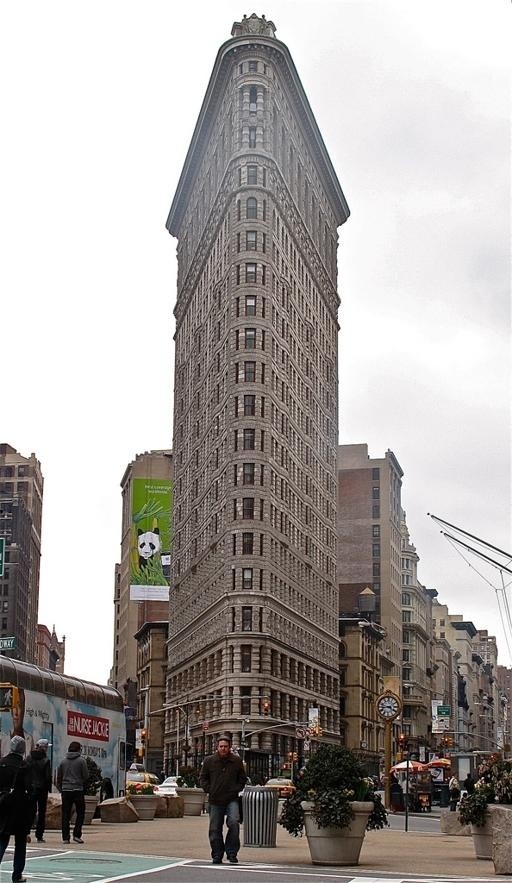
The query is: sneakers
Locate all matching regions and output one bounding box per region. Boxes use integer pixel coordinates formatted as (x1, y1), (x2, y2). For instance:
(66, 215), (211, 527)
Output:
(73, 836), (84, 843)
(213, 855), (222, 864)
(13, 878), (27, 883)
(27, 835), (31, 843)
(227, 852), (238, 863)
(37, 840), (45, 843)
(63, 840), (70, 844)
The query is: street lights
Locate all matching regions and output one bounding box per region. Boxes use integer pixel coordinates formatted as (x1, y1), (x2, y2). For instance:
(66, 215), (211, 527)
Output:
(241, 715), (251, 774)
(472, 698), (505, 752)
(402, 682), (417, 689)
(160, 701), (189, 766)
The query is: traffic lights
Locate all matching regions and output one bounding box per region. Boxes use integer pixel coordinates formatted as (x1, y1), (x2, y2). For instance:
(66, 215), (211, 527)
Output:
(292, 751), (299, 761)
(448, 736), (453, 746)
(399, 732), (406, 747)
(140, 730), (148, 745)
(138, 749), (145, 756)
(263, 697), (269, 715)
(317, 725), (323, 737)
(444, 736), (449, 746)
(288, 751), (292, 761)
(441, 737), (445, 745)
(0, 684), (19, 708)
(283, 762), (289, 768)
(403, 750), (410, 759)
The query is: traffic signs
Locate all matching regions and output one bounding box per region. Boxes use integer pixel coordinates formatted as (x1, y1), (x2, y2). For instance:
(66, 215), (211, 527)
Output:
(0, 635), (15, 652)
(239, 739), (249, 747)
(437, 704), (453, 715)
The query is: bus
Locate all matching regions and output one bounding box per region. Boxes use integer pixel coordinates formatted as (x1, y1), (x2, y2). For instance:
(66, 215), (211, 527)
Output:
(473, 749), (493, 758)
(0, 652), (130, 800)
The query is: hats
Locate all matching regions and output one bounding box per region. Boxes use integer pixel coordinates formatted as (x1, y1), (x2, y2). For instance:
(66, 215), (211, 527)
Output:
(68, 742), (81, 752)
(10, 735), (26, 753)
(36, 739), (53, 748)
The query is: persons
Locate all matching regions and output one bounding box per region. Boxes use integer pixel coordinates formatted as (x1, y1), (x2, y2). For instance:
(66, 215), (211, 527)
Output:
(0, 688), (34, 759)
(387, 772), (477, 813)
(200, 736), (247, 864)
(23, 739), (53, 844)
(0, 736), (33, 883)
(56, 741), (88, 844)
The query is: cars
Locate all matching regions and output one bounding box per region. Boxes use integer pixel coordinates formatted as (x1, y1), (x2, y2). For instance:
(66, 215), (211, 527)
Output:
(262, 774), (298, 798)
(361, 773), (389, 790)
(151, 774), (189, 796)
(123, 760), (161, 796)
(238, 776), (252, 797)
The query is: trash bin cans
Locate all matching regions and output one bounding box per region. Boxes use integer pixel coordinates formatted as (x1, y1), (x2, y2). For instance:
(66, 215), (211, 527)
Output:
(242, 786), (281, 849)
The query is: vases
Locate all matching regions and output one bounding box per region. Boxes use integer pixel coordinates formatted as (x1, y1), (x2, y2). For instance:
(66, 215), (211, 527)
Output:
(130, 795), (158, 820)
(301, 801), (373, 866)
(470, 804), (492, 860)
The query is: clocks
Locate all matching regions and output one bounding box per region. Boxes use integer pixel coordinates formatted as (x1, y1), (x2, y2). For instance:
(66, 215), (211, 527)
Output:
(376, 690), (402, 720)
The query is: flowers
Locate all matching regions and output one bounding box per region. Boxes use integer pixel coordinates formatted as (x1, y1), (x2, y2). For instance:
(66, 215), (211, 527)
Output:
(126, 782), (159, 795)
(279, 744), (391, 839)
(458, 759), (512, 827)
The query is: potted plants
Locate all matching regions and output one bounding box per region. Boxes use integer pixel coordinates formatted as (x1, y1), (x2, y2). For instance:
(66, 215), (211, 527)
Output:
(71, 756), (103, 824)
(175, 766), (205, 816)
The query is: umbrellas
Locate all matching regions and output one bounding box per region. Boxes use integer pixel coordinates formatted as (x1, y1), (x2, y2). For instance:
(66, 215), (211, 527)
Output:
(391, 760), (424, 777)
